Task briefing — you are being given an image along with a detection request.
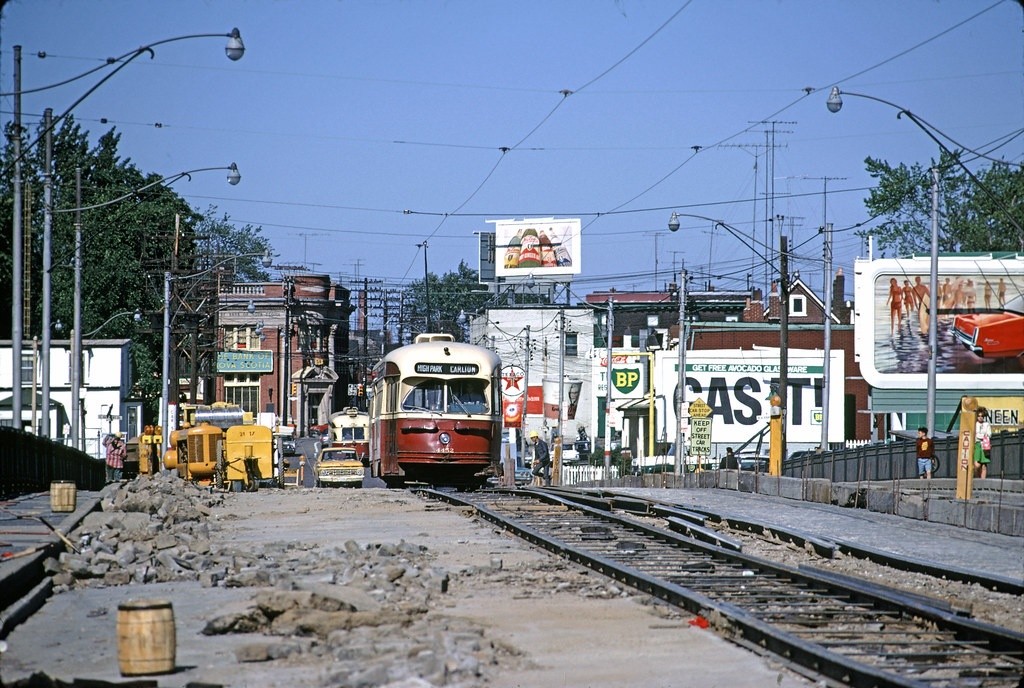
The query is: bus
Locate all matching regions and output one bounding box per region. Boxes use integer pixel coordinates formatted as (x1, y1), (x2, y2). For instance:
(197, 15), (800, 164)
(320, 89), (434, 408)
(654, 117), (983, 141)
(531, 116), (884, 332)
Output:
(329, 407), (371, 466)
(368, 333), (501, 487)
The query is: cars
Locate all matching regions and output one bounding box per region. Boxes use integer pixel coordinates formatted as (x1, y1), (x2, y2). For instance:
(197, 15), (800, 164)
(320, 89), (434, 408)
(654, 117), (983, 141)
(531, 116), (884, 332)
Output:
(314, 447), (365, 489)
(549, 444), (578, 463)
(280, 433), (295, 456)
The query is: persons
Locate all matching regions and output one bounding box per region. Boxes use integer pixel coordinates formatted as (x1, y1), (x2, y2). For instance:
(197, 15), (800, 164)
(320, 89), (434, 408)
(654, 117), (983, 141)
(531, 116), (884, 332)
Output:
(103, 432), (127, 482)
(719, 447), (738, 469)
(974, 410), (992, 479)
(886, 277), (1007, 336)
(459, 383), (484, 403)
(529, 432), (551, 487)
(915, 427), (935, 479)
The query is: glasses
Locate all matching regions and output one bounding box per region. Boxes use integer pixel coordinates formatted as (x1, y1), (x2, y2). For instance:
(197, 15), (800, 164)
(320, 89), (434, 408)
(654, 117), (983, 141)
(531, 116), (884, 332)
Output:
(977, 415), (984, 418)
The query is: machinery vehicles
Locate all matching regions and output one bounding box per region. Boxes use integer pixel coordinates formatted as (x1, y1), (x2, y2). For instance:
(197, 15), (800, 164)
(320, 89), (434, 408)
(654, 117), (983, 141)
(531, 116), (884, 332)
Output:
(163, 400), (283, 494)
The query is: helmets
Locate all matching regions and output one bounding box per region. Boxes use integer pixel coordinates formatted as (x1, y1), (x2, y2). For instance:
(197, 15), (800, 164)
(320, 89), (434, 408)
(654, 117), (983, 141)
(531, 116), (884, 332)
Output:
(115, 432), (122, 438)
(529, 431), (538, 438)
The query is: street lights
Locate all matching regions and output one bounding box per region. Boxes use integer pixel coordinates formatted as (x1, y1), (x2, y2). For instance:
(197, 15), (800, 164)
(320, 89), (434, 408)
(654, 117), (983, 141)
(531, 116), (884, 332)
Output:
(526, 274), (613, 465)
(13, 27), (244, 431)
(827, 86), (939, 440)
(459, 310), (530, 462)
(668, 210), (790, 473)
(41, 107), (241, 439)
(162, 249), (270, 476)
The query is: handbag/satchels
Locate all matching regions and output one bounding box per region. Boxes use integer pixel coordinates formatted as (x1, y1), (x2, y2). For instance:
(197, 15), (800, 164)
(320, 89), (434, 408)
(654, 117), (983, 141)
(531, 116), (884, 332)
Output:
(982, 435), (991, 450)
